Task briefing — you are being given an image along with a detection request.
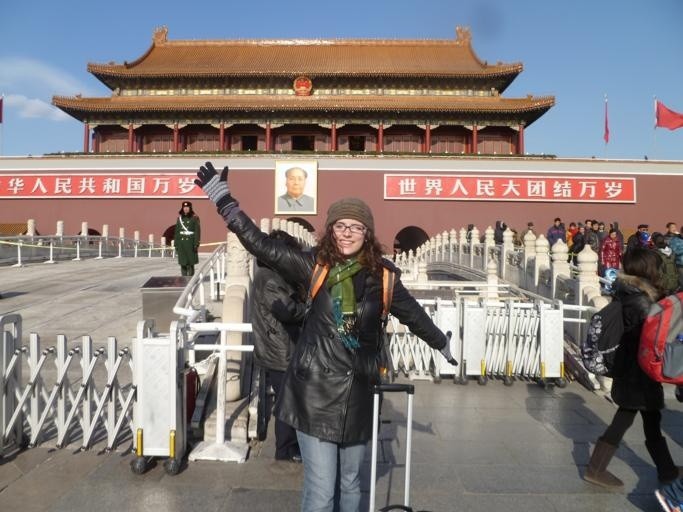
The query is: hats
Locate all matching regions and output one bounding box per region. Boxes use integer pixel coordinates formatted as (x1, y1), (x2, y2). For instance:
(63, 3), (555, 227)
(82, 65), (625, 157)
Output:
(182, 201), (192, 208)
(325, 198), (374, 233)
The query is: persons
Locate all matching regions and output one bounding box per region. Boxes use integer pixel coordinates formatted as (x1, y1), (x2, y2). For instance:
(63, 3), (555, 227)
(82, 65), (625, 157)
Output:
(489, 212), (682, 298)
(246, 228), (304, 463)
(580, 249), (683, 489)
(193, 158), (459, 510)
(173, 201), (201, 278)
(276, 165), (316, 212)
(466, 224), (477, 255)
(652, 382), (682, 512)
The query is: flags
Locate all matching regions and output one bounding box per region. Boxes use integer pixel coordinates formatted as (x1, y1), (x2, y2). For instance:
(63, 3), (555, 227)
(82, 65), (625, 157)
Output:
(602, 97), (609, 145)
(654, 99), (683, 130)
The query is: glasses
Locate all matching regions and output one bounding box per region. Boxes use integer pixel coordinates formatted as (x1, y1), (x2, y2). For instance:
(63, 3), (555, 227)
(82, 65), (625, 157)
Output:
(332, 222), (368, 233)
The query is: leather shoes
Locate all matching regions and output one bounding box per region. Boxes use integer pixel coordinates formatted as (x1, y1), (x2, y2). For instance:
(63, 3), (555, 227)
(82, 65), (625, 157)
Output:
(275, 448), (302, 464)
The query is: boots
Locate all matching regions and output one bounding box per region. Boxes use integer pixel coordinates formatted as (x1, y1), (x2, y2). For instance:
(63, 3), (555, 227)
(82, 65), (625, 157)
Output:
(645, 436), (683, 485)
(583, 437), (624, 488)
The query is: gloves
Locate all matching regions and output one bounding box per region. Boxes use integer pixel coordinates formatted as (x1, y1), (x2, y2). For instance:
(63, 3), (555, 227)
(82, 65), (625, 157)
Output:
(444, 331), (457, 367)
(195, 162), (237, 214)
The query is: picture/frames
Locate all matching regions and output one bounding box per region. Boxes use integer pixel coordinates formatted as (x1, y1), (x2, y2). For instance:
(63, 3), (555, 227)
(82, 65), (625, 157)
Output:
(274, 160), (318, 216)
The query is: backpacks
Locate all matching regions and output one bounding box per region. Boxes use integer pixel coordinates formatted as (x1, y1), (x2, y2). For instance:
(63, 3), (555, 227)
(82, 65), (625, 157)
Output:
(581, 301), (625, 377)
(638, 292), (683, 385)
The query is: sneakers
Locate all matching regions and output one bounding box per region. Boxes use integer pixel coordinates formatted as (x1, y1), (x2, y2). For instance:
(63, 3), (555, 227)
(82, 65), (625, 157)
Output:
(654, 488), (683, 512)
